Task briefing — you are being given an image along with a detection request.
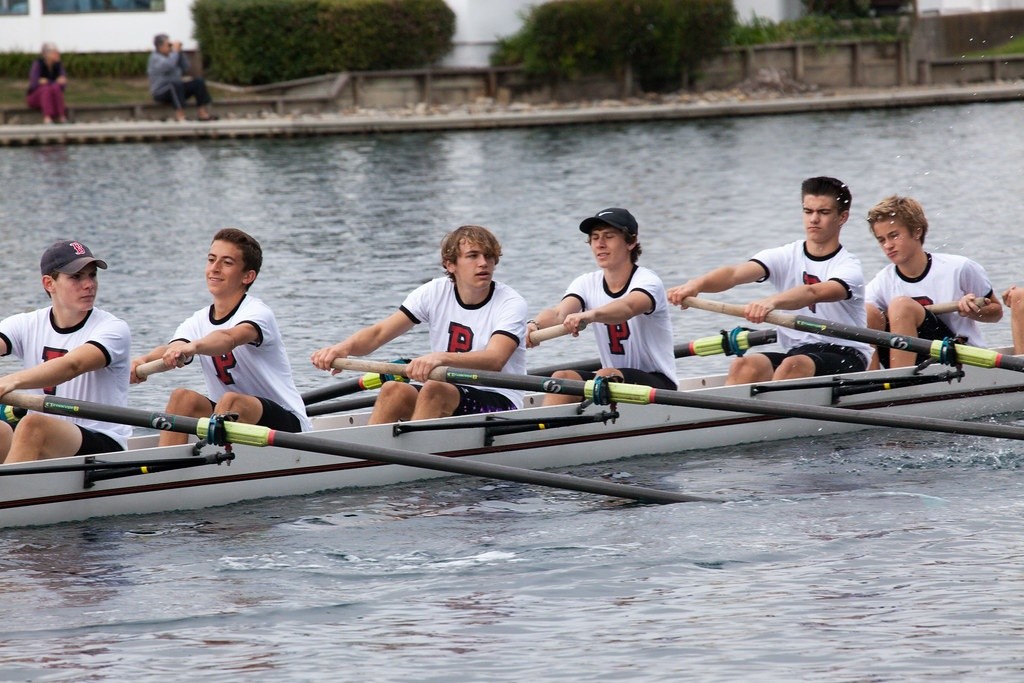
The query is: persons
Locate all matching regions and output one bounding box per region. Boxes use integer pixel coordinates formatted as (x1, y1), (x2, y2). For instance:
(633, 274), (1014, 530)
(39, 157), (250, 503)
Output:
(1001, 283), (1024, 356)
(863, 195), (1003, 368)
(667, 176), (876, 385)
(24, 43), (68, 126)
(525, 207), (678, 407)
(129, 228), (310, 447)
(148, 34), (222, 122)
(0, 241), (130, 463)
(312, 227), (526, 426)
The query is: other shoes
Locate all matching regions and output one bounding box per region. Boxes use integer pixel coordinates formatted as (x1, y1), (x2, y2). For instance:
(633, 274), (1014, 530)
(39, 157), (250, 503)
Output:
(198, 115), (219, 121)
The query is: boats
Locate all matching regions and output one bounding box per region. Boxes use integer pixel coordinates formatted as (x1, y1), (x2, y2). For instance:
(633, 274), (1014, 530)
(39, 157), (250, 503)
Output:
(1, 339), (1024, 534)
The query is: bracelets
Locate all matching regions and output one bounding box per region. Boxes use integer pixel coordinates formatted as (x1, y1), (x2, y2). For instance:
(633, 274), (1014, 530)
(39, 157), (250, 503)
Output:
(526, 318), (540, 330)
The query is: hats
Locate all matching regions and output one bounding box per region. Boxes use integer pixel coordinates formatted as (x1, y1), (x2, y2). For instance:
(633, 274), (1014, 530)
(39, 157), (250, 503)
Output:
(579, 208), (639, 238)
(40, 240), (108, 276)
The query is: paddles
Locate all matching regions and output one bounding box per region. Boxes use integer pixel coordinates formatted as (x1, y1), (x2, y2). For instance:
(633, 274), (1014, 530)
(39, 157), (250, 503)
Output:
(304, 296), (1024, 418)
(305, 296), (987, 359)
(300, 318), (588, 406)
(0, 353), (186, 424)
(329, 357), (1024, 440)
(0, 391), (725, 504)
